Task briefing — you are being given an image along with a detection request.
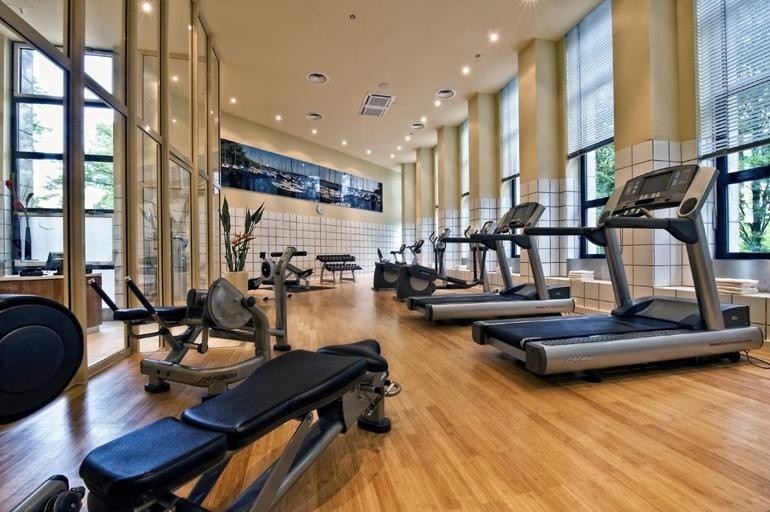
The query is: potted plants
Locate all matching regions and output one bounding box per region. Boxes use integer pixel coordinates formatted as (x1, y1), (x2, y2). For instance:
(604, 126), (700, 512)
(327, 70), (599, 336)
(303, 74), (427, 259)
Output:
(217, 194), (265, 295)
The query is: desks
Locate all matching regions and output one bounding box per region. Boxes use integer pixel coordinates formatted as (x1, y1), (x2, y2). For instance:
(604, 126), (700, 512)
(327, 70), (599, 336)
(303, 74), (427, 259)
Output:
(0, 272), (103, 335)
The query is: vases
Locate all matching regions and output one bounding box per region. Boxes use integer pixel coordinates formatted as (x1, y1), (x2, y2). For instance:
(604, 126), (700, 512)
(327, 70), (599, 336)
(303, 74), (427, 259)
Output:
(25, 226), (32, 260)
(11, 214), (22, 260)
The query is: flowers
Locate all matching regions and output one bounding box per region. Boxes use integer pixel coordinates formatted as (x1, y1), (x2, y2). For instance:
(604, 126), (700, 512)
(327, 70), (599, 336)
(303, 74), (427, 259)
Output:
(5, 172), (35, 228)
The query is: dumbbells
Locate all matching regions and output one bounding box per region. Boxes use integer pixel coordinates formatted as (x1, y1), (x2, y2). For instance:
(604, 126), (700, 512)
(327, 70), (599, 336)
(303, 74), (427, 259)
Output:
(325, 263), (361, 272)
(317, 255), (355, 262)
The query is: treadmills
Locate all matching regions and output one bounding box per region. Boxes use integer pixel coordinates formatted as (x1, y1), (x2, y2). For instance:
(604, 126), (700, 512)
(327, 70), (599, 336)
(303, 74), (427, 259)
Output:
(407, 201), (575, 325)
(472, 165), (764, 383)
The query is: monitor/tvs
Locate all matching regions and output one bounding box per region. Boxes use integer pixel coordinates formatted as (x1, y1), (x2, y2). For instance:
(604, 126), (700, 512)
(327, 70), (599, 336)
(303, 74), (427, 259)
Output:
(511, 204), (529, 218)
(635, 167), (677, 195)
(45, 251), (64, 275)
(482, 221), (492, 230)
